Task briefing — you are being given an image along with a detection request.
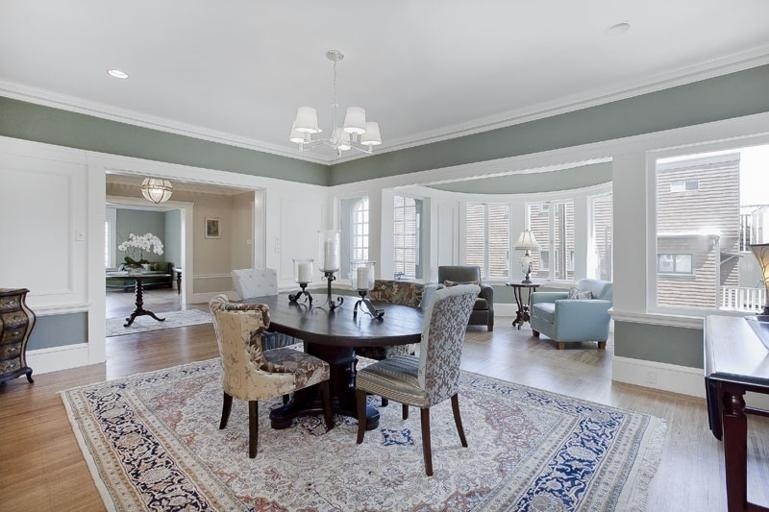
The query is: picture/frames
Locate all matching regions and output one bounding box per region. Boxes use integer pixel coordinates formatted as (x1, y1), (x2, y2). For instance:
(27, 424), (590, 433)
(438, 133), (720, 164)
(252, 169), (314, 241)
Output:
(656, 253), (693, 274)
(206, 218), (221, 238)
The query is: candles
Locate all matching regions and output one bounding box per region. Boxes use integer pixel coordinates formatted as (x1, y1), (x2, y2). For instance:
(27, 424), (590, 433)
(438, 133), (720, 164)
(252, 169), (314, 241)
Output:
(357, 266), (370, 290)
(324, 239), (340, 273)
(298, 262), (313, 284)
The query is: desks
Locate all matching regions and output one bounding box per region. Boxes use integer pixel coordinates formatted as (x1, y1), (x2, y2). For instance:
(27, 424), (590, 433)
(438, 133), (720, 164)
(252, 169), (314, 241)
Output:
(704, 314), (768, 512)
(106, 271), (177, 326)
(237, 296), (423, 429)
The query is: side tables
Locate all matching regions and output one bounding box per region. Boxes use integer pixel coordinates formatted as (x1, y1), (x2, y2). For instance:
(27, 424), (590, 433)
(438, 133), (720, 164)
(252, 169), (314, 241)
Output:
(506, 282), (541, 330)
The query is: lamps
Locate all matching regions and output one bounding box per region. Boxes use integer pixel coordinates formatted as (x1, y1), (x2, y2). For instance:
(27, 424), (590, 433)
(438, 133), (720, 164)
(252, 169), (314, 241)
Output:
(751, 241), (768, 323)
(141, 175), (172, 203)
(514, 229), (541, 284)
(290, 48), (383, 158)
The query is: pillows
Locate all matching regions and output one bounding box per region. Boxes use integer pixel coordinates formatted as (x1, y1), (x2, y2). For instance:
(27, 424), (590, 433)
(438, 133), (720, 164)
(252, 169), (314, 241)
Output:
(444, 277), (482, 288)
(568, 285), (596, 300)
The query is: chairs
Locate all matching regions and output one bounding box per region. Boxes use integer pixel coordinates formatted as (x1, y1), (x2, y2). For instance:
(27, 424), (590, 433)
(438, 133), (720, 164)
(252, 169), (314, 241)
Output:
(209, 292), (332, 456)
(355, 284), (481, 479)
(231, 268), (304, 406)
(529, 276), (613, 352)
(438, 267), (494, 332)
(353, 279), (425, 408)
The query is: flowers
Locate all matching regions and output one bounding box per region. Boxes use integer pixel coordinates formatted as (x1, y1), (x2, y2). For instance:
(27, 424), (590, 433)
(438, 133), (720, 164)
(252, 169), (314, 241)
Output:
(117, 230), (166, 256)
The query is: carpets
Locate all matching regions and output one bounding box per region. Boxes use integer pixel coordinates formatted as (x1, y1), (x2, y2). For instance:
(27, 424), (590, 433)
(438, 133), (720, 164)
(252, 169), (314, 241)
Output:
(106, 309), (215, 337)
(57, 341), (668, 512)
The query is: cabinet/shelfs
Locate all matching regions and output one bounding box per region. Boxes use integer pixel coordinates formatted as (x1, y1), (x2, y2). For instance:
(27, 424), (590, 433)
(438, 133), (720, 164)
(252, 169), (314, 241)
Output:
(0, 288), (37, 393)
